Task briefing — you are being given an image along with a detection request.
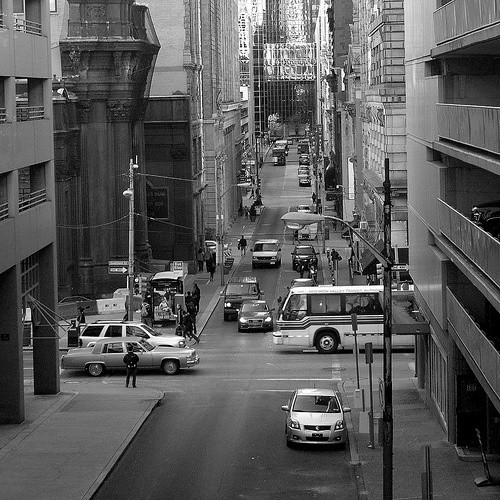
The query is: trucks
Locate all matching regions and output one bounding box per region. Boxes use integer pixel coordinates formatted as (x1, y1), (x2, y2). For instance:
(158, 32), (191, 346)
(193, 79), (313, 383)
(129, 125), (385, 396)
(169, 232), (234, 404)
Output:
(150, 271), (197, 322)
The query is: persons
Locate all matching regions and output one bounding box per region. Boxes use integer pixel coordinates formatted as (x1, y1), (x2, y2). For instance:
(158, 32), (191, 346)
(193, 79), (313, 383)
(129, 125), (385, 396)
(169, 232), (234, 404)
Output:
(134, 282), (202, 344)
(196, 247), (216, 282)
(79, 308), (86, 323)
(318, 203), (322, 214)
(311, 192), (317, 204)
(244, 205), (250, 219)
(331, 249), (342, 270)
(238, 236), (247, 256)
(123, 346), (139, 389)
(332, 216), (338, 231)
(319, 171), (322, 180)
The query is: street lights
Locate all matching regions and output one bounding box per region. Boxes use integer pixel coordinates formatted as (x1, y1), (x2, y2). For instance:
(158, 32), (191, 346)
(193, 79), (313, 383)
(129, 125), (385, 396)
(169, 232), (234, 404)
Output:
(123, 189), (134, 321)
(221, 182), (251, 285)
(281, 211), (392, 500)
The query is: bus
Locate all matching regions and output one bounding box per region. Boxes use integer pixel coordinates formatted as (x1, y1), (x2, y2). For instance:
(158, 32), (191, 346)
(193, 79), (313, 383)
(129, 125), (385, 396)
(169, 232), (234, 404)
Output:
(272, 140), (288, 166)
(272, 286), (416, 354)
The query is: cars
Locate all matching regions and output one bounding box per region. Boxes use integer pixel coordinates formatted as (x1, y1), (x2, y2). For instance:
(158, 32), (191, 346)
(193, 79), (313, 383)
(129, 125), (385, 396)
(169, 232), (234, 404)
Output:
(297, 143), (312, 186)
(62, 321), (200, 374)
(281, 388), (351, 450)
(292, 245), (320, 271)
(204, 240), (228, 252)
(220, 276), (273, 332)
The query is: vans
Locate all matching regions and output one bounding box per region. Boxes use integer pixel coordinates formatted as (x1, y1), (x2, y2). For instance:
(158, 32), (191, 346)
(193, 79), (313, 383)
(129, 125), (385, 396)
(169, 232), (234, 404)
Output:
(250, 239), (281, 268)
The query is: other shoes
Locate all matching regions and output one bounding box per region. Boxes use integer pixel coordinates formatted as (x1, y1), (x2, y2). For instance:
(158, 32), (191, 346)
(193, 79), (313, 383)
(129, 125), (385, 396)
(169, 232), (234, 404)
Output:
(197, 310), (199, 312)
(189, 337), (192, 341)
(194, 332), (196, 334)
(126, 385), (128, 387)
(197, 339), (200, 344)
(133, 385), (136, 388)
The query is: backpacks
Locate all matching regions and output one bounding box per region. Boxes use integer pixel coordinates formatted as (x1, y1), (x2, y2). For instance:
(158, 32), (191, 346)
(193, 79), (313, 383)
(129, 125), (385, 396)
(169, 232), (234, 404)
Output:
(141, 303), (149, 317)
